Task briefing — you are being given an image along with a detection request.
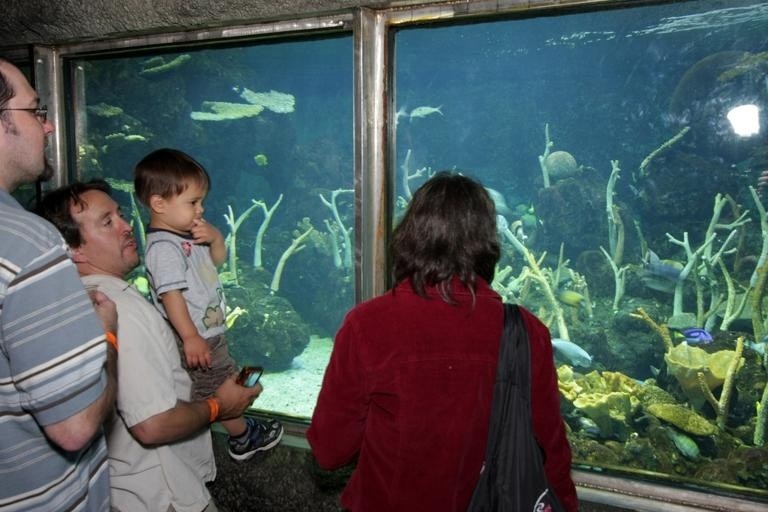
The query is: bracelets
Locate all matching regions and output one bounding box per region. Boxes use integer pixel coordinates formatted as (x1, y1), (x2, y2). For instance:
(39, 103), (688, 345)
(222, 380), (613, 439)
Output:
(105, 329), (119, 353)
(206, 400), (219, 423)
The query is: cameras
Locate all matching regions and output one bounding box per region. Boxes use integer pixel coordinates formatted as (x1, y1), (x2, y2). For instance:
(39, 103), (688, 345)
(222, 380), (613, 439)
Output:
(237, 366), (263, 386)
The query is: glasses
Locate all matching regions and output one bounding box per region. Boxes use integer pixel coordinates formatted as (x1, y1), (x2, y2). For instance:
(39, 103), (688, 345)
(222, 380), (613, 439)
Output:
(0, 105), (47, 123)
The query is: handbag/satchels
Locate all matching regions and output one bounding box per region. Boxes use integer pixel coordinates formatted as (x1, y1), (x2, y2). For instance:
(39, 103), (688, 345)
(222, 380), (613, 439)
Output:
(465, 302), (564, 512)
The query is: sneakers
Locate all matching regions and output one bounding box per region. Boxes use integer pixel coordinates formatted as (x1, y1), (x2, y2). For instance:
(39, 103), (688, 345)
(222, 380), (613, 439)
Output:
(226, 414), (285, 461)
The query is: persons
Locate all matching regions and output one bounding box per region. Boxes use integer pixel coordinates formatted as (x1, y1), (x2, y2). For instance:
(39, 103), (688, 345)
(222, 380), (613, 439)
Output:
(134, 148), (287, 465)
(305, 168), (579, 512)
(0, 58), (119, 511)
(23, 178), (264, 510)
